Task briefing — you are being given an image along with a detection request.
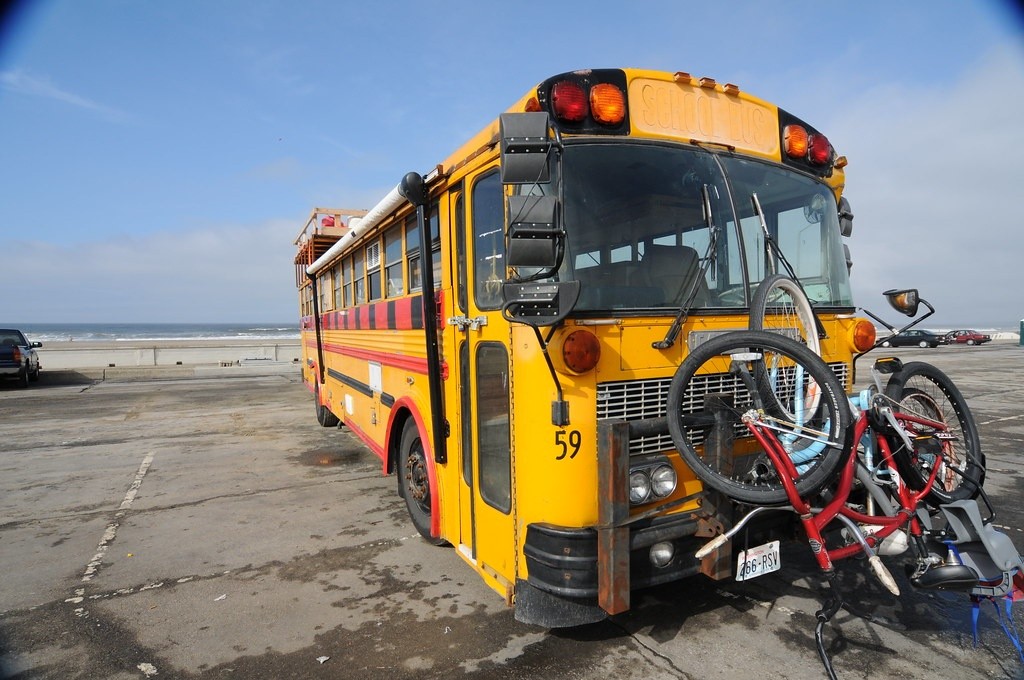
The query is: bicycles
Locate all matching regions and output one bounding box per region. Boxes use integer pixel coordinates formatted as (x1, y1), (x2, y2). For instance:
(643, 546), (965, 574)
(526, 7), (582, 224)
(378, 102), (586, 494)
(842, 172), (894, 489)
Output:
(669, 271), (1023, 679)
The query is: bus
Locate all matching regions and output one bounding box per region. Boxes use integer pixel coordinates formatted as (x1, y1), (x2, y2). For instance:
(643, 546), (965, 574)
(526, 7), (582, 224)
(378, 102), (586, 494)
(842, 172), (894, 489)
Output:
(294, 66), (875, 627)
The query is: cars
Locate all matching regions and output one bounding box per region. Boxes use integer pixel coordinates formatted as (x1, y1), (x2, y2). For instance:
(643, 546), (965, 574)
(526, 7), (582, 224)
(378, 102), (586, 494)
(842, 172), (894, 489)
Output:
(0, 329), (43, 387)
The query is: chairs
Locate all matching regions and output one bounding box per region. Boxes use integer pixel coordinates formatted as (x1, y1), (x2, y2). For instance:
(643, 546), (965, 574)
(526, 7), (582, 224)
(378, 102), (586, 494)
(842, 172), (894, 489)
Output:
(637, 244), (714, 307)
(4, 338), (15, 347)
